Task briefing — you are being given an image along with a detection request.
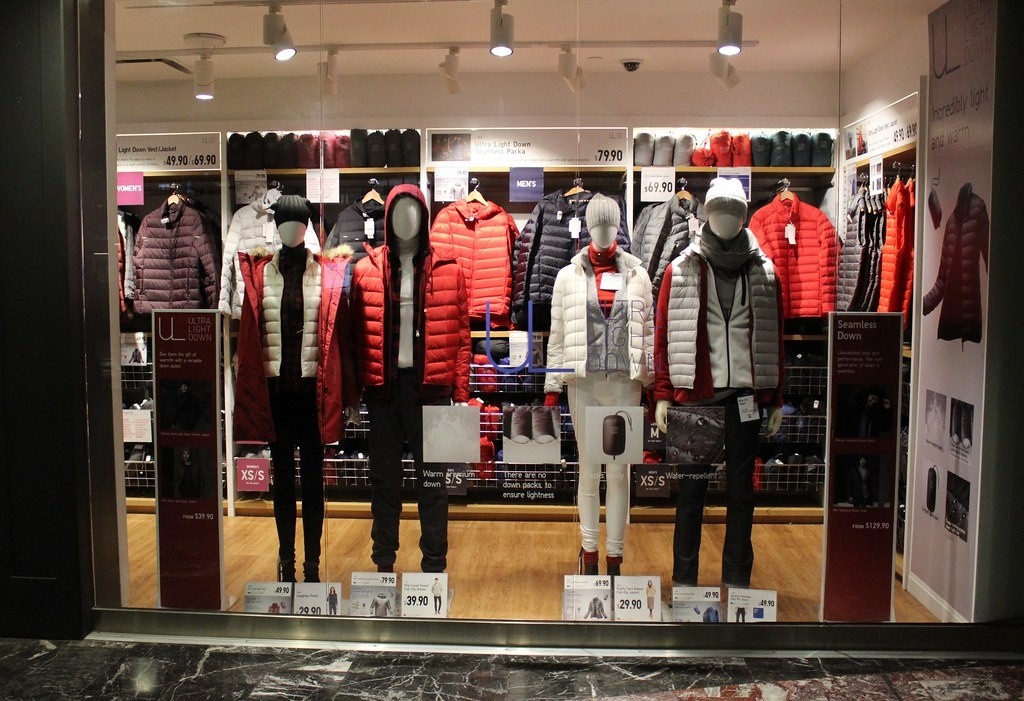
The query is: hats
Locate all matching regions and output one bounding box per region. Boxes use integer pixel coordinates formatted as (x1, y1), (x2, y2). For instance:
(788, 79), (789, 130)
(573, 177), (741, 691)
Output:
(270, 195), (310, 230)
(703, 177), (748, 219)
(584, 193), (621, 233)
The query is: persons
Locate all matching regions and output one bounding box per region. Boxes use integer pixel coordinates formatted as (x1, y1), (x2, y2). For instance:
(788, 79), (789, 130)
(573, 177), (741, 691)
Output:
(544, 193), (654, 577)
(230, 195), (350, 584)
(654, 176), (784, 595)
(431, 577), (444, 615)
(327, 587), (339, 615)
(646, 579), (656, 619)
(344, 184), (473, 574)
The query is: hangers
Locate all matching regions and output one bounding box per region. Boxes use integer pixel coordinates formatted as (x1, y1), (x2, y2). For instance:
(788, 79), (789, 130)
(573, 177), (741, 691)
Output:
(677, 178), (691, 201)
(770, 177), (793, 200)
(467, 178), (489, 207)
(167, 185), (195, 206)
(266, 181), (284, 201)
(563, 177), (590, 197)
(362, 178), (384, 206)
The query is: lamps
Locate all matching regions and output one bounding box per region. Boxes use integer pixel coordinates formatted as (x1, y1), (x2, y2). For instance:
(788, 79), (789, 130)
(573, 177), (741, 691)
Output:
(709, 47), (741, 91)
(182, 33), (227, 100)
(558, 46), (585, 92)
(438, 47), (465, 94)
(717, 0), (743, 55)
(317, 49), (340, 96)
(262, 4), (298, 63)
(489, 0), (516, 58)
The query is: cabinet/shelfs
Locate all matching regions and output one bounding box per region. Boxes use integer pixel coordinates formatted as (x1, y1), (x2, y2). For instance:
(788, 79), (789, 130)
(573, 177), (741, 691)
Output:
(846, 140), (917, 576)
(121, 125), (836, 523)
